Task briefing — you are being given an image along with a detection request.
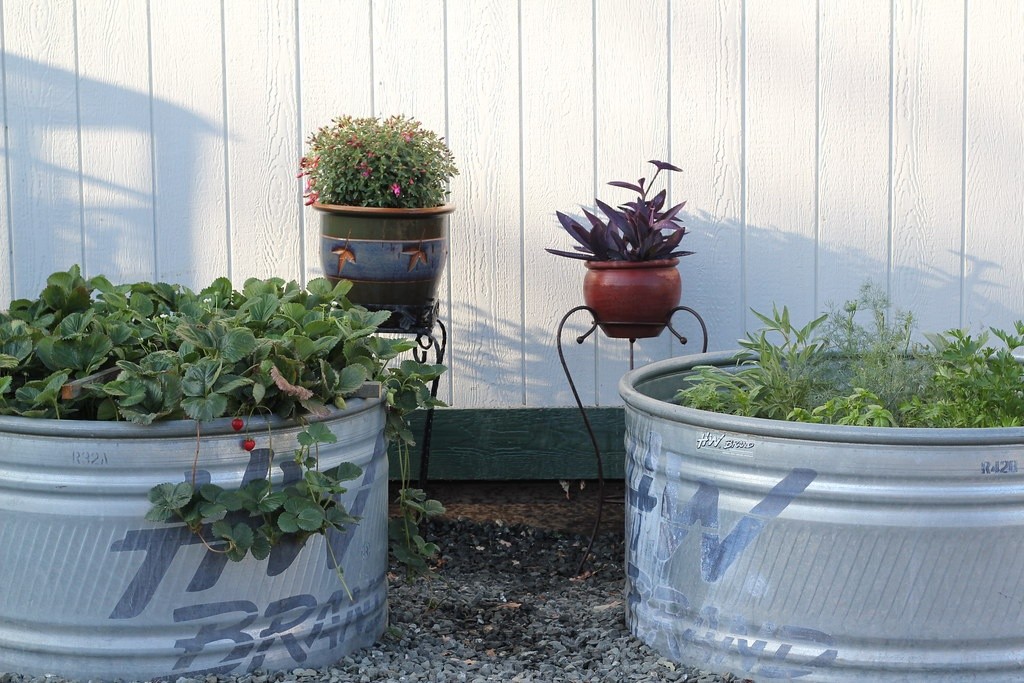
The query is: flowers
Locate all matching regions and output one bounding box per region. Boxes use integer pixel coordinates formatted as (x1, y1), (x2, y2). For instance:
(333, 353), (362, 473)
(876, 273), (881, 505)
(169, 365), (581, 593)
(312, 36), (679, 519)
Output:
(295, 111), (460, 209)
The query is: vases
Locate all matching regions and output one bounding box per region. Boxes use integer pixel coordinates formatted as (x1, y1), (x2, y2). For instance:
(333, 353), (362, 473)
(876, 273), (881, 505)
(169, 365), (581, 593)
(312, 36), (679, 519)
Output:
(311, 203), (457, 324)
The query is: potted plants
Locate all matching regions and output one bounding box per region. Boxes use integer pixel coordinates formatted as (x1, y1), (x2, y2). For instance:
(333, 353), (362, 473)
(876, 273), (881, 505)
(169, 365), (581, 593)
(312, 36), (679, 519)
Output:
(545, 159), (697, 338)
(616, 274), (1024, 683)
(0, 261), (455, 683)
